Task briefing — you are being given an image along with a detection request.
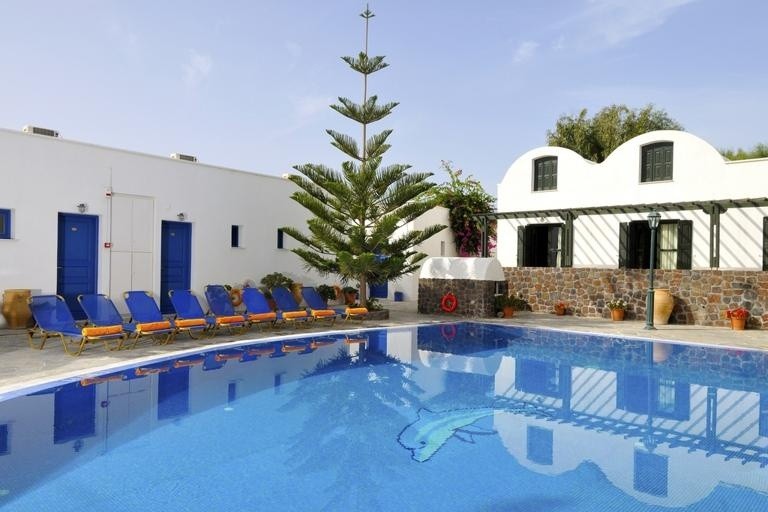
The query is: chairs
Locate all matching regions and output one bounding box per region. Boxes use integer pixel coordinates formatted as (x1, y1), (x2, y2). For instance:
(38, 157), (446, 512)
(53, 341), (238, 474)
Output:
(75, 294), (176, 351)
(121, 289), (208, 345)
(168, 287), (245, 341)
(238, 288), (309, 328)
(270, 288), (337, 327)
(26, 294), (128, 357)
(299, 287), (368, 327)
(202, 286), (278, 334)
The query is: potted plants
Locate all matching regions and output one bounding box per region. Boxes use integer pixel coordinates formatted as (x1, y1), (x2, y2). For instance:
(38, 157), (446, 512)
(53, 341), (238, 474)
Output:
(497, 294), (519, 318)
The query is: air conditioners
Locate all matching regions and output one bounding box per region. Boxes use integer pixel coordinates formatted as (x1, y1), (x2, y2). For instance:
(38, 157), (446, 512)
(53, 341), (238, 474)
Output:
(169, 153), (197, 162)
(282, 173), (302, 181)
(22, 125), (59, 138)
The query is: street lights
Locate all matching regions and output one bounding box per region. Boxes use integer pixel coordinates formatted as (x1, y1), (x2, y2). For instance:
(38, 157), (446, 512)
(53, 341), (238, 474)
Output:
(643, 209), (662, 330)
(640, 340), (659, 453)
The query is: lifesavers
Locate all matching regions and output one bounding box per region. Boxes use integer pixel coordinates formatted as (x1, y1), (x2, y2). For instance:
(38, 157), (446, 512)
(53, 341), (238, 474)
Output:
(229, 288), (242, 306)
(441, 322), (458, 340)
(441, 293), (458, 312)
(331, 286), (341, 298)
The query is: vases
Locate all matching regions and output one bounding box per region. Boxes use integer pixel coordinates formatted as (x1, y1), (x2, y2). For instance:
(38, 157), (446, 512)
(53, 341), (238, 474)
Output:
(731, 317), (745, 330)
(557, 309), (564, 315)
(611, 309), (624, 321)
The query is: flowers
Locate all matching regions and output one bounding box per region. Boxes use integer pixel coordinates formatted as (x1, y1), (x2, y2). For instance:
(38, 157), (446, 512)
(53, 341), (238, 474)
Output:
(606, 298), (627, 312)
(726, 306), (749, 319)
(554, 300), (567, 310)
